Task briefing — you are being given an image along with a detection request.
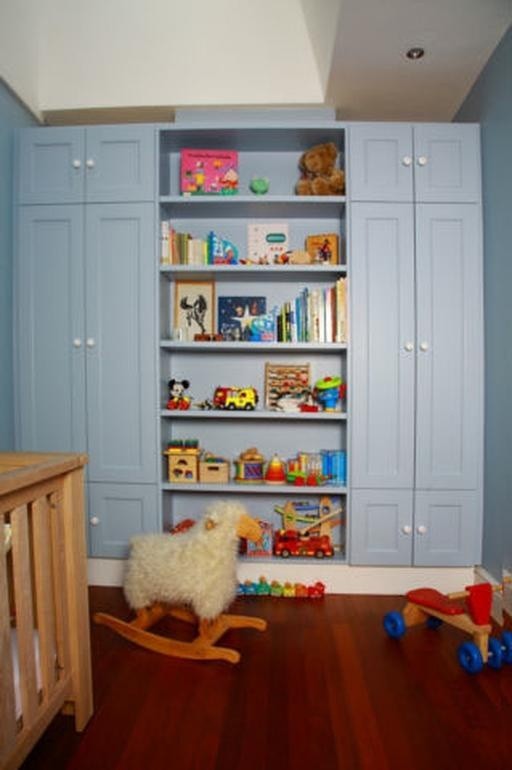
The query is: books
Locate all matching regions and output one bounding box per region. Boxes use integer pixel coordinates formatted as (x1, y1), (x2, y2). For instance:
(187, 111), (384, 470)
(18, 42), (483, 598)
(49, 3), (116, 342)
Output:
(160, 221), (348, 342)
(179, 147), (239, 196)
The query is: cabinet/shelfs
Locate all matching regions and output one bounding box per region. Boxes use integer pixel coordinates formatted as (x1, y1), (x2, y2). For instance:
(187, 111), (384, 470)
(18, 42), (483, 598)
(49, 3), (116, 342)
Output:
(13, 124), (161, 561)
(157, 127), (347, 565)
(344, 120), (484, 567)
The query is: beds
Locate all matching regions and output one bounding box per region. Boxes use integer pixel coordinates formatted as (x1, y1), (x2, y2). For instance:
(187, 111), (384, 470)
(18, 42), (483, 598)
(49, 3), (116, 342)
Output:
(0, 450), (94, 770)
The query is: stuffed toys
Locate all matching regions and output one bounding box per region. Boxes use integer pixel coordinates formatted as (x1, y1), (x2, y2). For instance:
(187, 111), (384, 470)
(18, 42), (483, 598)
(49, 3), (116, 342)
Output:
(296, 141), (345, 195)
